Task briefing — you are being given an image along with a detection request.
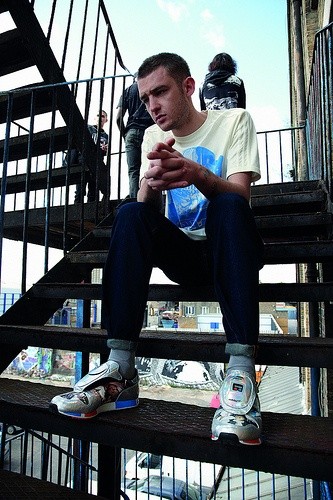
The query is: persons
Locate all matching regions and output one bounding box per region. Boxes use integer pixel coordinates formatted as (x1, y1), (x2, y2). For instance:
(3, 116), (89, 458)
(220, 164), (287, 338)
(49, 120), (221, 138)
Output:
(63, 52), (246, 204)
(48, 52), (263, 445)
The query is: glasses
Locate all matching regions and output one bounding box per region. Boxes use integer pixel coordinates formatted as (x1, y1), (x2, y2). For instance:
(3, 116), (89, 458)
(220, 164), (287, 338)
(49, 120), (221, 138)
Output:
(97, 114), (105, 118)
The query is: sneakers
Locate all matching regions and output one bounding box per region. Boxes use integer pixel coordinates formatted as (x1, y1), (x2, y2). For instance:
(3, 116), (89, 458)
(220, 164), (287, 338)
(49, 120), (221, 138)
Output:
(49, 361), (140, 421)
(211, 370), (263, 448)
